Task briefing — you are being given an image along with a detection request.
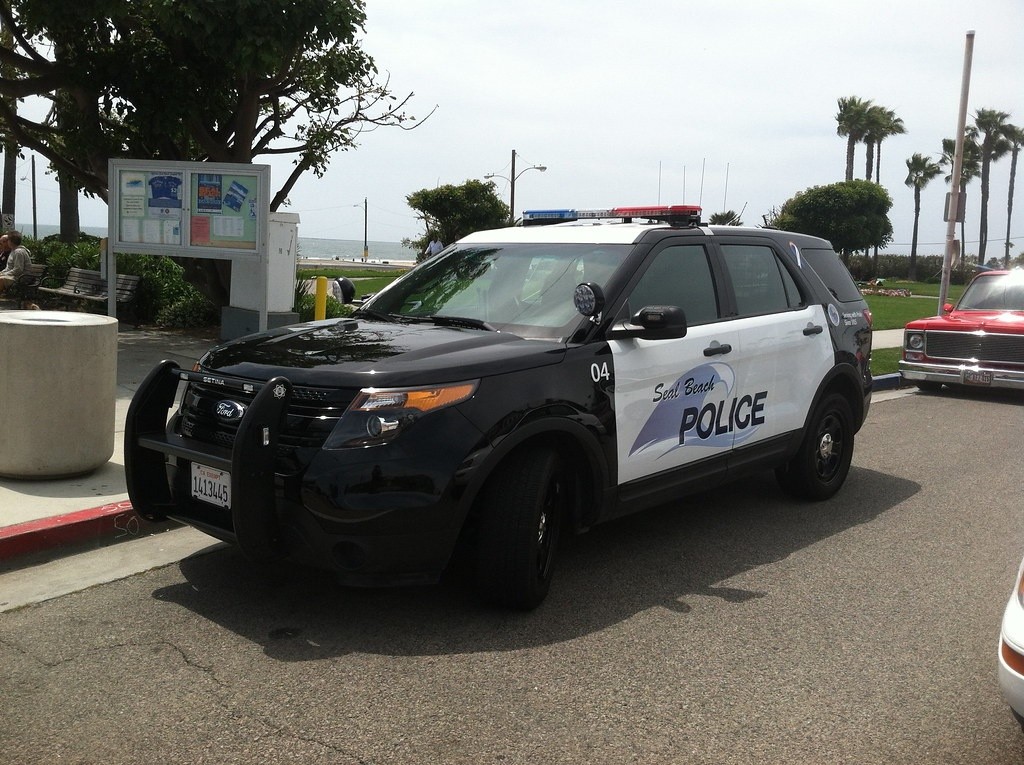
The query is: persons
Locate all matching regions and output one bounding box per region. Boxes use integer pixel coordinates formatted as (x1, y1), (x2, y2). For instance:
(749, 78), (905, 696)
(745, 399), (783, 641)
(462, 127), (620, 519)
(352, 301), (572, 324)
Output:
(455, 234), (460, 242)
(0, 231), (33, 290)
(424, 235), (444, 281)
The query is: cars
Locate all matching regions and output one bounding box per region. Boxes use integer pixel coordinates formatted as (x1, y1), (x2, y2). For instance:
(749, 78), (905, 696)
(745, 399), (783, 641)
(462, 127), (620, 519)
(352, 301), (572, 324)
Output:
(897, 269), (1024, 390)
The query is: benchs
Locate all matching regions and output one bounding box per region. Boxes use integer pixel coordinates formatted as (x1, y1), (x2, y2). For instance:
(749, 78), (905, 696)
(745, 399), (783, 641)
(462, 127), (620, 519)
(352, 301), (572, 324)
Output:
(2, 264), (48, 310)
(34, 266), (145, 330)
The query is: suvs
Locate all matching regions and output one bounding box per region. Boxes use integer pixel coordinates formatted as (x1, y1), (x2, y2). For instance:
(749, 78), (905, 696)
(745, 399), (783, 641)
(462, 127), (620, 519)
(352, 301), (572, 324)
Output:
(123, 201), (872, 616)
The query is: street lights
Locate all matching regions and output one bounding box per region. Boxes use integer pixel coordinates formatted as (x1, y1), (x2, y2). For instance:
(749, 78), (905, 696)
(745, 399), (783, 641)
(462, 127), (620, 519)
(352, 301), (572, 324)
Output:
(483, 150), (547, 227)
(354, 196), (368, 263)
